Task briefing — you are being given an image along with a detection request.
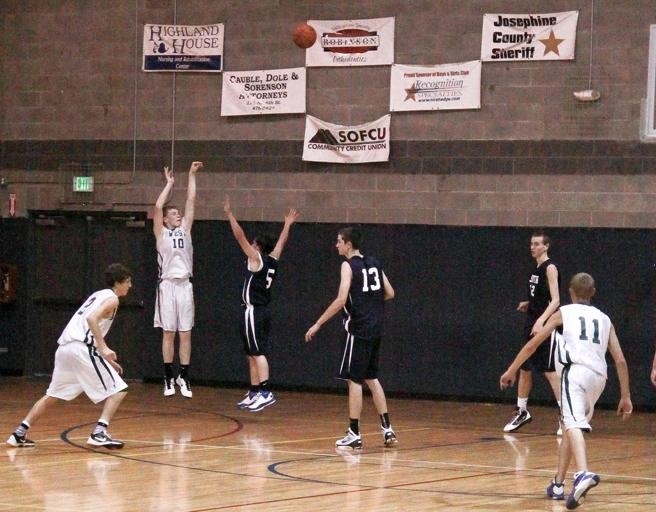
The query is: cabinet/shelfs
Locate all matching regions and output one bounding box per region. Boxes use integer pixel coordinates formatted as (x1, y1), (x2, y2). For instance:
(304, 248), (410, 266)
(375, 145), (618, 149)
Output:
(0, 217), (27, 377)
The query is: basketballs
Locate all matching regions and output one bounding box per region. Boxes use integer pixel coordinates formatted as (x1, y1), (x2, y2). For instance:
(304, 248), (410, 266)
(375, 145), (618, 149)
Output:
(293, 25), (316, 49)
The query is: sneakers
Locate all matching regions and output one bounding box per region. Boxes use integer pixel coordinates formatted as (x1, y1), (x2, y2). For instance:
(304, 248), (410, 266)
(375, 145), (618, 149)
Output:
(336, 427), (362, 449)
(176, 374), (192, 399)
(6, 432), (35, 448)
(503, 406), (532, 433)
(545, 420), (600, 511)
(87, 430), (124, 450)
(381, 424), (397, 448)
(236, 390), (261, 410)
(163, 376), (175, 397)
(247, 391), (280, 413)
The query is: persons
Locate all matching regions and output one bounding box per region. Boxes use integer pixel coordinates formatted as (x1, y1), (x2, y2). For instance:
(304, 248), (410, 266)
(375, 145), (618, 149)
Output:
(502, 228), (563, 437)
(153, 161), (205, 399)
(499, 273), (633, 509)
(6, 263), (134, 448)
(221, 193), (300, 412)
(304, 226), (399, 450)
(650, 354), (656, 386)
